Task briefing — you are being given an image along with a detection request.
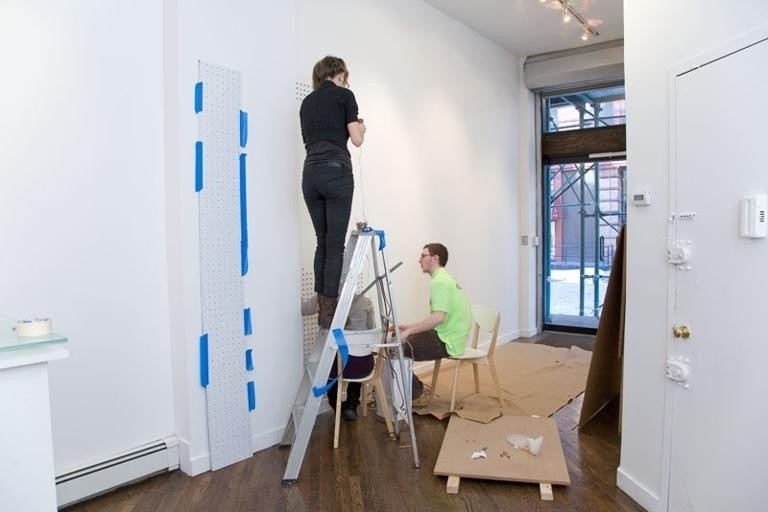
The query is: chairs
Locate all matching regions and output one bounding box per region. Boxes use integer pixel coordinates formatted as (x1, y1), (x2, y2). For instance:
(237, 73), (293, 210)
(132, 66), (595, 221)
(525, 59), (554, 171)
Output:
(333, 302), (505, 451)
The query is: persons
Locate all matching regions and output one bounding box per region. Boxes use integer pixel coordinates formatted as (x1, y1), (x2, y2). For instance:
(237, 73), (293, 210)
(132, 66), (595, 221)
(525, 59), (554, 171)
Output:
(301, 292), (375, 420)
(300, 55), (366, 328)
(389, 244), (471, 401)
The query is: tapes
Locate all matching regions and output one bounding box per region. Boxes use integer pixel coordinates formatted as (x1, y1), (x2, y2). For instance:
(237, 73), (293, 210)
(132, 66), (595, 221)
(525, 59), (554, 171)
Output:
(15, 317), (51, 337)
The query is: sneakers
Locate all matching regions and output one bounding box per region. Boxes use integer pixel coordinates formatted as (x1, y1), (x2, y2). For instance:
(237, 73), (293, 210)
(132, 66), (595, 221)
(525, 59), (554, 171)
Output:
(342, 409), (358, 421)
(412, 393), (429, 409)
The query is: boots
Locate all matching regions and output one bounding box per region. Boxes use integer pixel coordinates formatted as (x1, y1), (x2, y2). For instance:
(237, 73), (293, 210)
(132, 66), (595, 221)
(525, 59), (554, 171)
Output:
(317, 295), (351, 329)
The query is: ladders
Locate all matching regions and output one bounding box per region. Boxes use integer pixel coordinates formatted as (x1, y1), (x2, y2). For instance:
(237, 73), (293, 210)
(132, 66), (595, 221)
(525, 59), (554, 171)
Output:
(278, 230), (420, 486)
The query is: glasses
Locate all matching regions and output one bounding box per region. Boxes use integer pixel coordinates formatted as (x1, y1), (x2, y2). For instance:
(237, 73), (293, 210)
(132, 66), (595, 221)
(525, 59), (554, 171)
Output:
(344, 78), (350, 89)
(421, 253), (434, 257)
